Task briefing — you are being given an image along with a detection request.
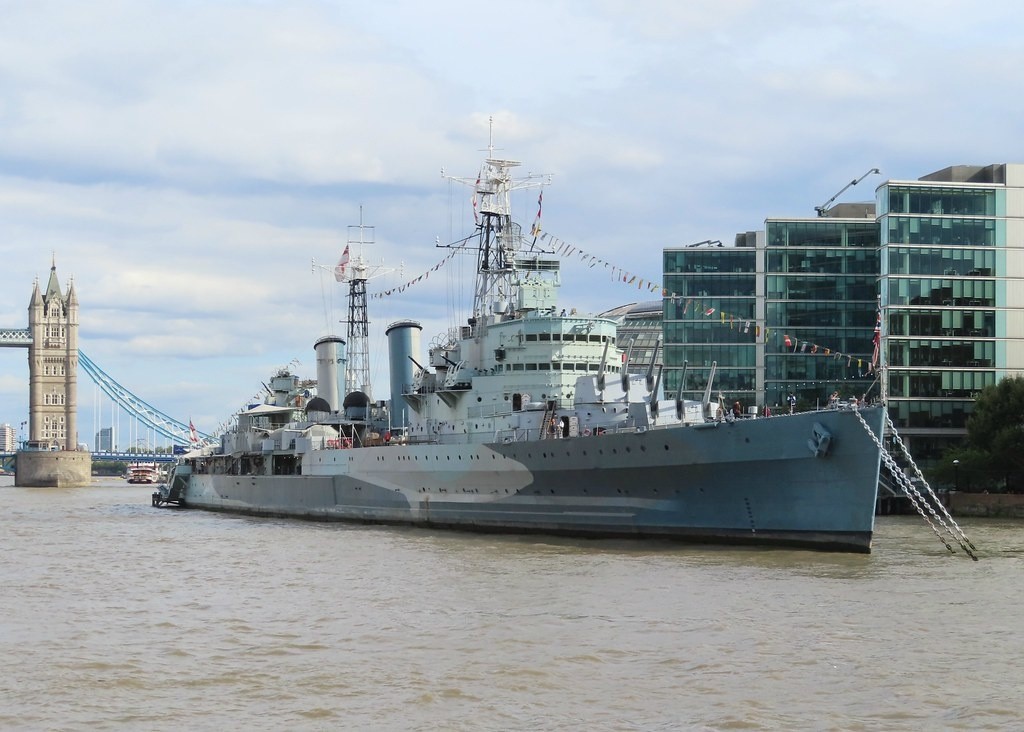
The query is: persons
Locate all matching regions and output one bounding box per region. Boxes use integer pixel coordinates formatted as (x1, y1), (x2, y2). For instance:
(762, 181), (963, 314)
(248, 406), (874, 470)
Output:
(734, 399), (742, 418)
(548, 418), (559, 442)
(762, 406), (772, 416)
(829, 389), (840, 403)
(786, 391), (796, 412)
(384, 429), (392, 446)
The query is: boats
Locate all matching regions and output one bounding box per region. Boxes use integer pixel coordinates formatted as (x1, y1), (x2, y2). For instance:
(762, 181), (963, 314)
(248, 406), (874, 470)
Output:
(149, 113), (886, 554)
(125, 460), (162, 483)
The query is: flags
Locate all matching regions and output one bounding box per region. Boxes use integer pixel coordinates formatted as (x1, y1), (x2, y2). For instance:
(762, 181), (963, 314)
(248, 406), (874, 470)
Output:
(331, 244), (353, 283)
(469, 172), (485, 225)
(871, 311), (882, 366)
(189, 420), (205, 443)
(530, 190), (546, 249)
